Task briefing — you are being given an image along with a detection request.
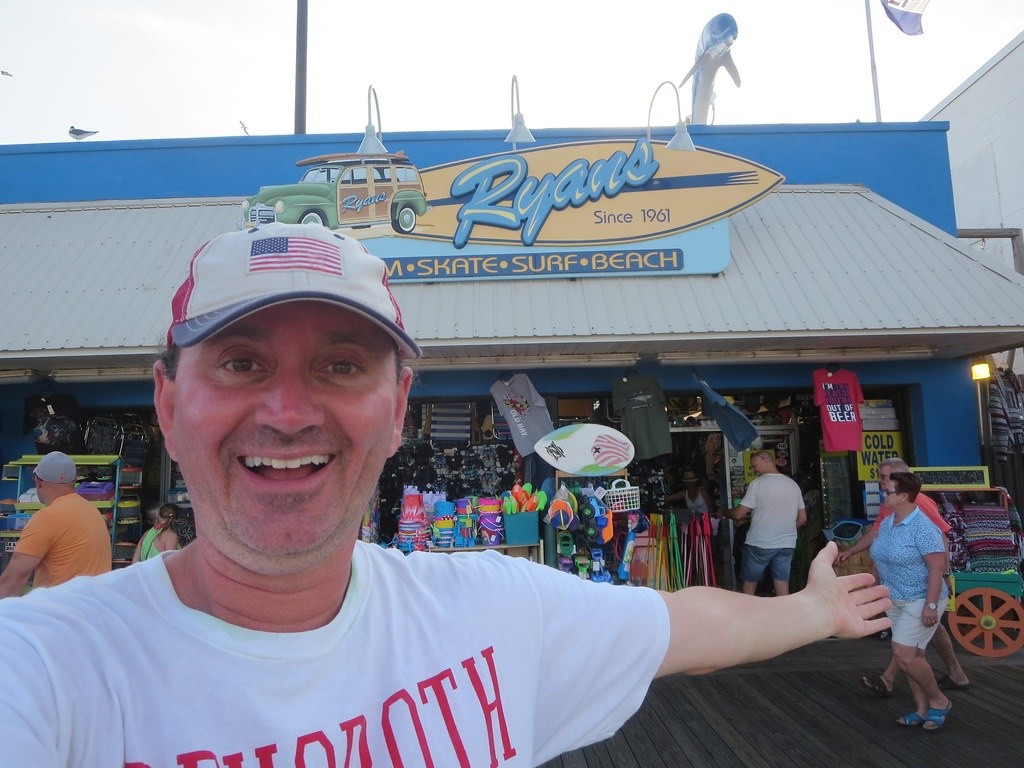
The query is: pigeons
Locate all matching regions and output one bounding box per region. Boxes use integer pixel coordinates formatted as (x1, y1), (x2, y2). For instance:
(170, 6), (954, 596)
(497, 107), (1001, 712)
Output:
(68, 126), (100, 142)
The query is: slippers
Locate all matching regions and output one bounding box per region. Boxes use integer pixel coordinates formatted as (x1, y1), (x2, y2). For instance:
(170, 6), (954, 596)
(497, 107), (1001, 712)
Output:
(937, 676), (972, 691)
(858, 676), (893, 698)
(895, 712), (926, 727)
(923, 701), (952, 730)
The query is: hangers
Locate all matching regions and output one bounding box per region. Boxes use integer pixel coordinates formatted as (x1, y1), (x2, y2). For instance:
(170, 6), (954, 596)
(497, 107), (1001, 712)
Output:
(494, 368), (514, 382)
(691, 365), (702, 381)
(818, 356), (842, 373)
(621, 367), (640, 380)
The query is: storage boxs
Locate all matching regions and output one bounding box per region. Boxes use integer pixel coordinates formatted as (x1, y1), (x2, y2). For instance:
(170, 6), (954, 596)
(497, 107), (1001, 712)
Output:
(6, 513), (32, 531)
(76, 481), (115, 501)
(503, 510), (539, 545)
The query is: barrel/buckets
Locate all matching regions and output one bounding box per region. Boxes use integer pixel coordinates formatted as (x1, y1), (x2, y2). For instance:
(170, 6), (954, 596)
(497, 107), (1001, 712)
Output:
(398, 493), (504, 552)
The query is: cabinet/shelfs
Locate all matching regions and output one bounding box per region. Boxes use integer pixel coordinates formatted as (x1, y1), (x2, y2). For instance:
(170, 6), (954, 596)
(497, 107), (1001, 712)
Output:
(0, 452), (142, 580)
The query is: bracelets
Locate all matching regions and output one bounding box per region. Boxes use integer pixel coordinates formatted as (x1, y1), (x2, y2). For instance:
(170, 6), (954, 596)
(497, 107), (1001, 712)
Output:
(943, 572), (951, 578)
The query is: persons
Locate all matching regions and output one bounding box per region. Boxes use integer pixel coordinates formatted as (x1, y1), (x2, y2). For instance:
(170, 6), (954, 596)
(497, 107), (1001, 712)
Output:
(0, 452), (113, 599)
(132, 504), (181, 564)
(0, 222), (893, 768)
(725, 451), (808, 596)
(665, 471), (712, 514)
(870, 472), (952, 729)
(835, 458), (971, 695)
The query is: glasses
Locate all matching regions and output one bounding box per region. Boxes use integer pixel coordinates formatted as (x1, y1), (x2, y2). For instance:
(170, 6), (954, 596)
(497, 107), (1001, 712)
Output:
(750, 453), (766, 460)
(886, 489), (911, 496)
(877, 473), (890, 480)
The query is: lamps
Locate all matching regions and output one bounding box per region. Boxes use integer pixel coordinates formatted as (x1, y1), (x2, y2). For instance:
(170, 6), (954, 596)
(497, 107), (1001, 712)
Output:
(504, 75), (536, 152)
(356, 85), (389, 156)
(645, 81), (695, 154)
(972, 354), (990, 384)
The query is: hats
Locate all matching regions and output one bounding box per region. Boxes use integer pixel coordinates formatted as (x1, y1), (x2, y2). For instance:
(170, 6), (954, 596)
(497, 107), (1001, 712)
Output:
(681, 471), (699, 482)
(167, 222), (423, 359)
(33, 452), (76, 483)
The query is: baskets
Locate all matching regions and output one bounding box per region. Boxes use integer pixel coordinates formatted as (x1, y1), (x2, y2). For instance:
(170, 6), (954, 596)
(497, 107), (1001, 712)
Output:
(604, 479), (641, 513)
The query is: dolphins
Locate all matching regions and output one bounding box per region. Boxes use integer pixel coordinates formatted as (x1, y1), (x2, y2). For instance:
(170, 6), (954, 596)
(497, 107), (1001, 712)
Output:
(679, 13), (741, 124)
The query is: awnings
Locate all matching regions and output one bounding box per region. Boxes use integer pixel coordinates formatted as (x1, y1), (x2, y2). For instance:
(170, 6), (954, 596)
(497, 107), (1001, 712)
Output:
(0, 185), (1024, 356)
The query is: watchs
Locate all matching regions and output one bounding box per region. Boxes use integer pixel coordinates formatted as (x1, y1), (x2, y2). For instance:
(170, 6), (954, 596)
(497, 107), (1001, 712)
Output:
(925, 603), (938, 610)
